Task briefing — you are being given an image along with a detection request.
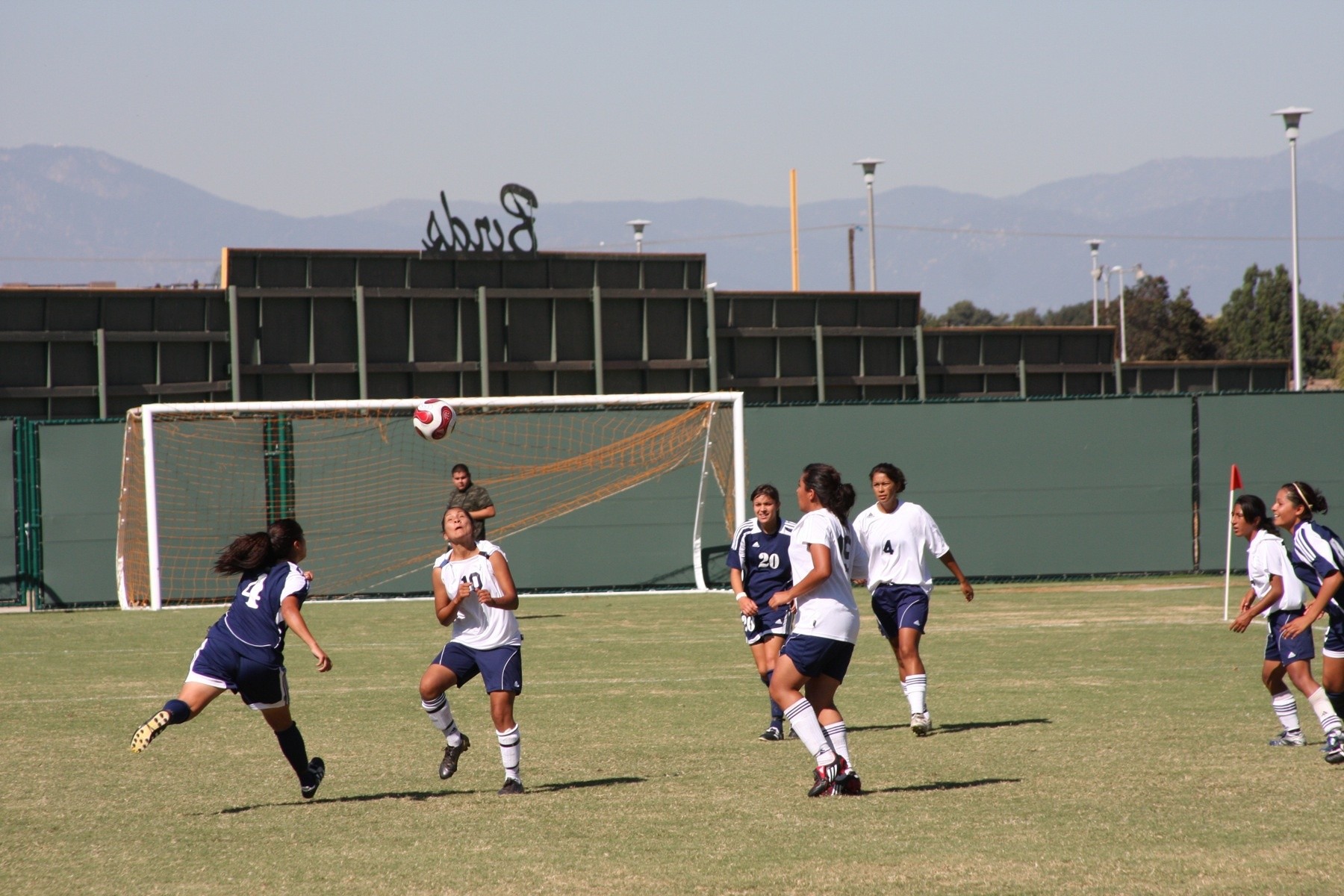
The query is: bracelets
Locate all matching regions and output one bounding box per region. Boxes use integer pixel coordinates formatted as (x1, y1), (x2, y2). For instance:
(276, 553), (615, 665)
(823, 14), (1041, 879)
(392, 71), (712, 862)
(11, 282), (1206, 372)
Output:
(736, 592), (746, 600)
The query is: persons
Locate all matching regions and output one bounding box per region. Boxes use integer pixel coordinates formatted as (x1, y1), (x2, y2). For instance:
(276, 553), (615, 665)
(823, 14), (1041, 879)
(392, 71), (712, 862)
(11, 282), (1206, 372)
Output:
(447, 463), (496, 554)
(1228, 494), (1344, 752)
(1271, 481), (1344, 763)
(132, 520), (333, 799)
(768, 462), (861, 796)
(726, 484), (799, 742)
(418, 507), (525, 795)
(852, 463), (974, 736)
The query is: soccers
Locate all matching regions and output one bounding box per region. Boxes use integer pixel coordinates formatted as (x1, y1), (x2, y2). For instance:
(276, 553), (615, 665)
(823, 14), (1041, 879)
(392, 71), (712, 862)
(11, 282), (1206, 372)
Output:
(412, 398), (457, 441)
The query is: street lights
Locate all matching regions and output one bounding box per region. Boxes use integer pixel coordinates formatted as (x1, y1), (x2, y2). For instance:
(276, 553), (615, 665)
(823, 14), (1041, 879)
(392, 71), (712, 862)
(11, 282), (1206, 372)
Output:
(626, 219), (652, 252)
(1092, 262), (1145, 363)
(854, 158), (884, 290)
(1086, 236), (1102, 323)
(1272, 106), (1316, 389)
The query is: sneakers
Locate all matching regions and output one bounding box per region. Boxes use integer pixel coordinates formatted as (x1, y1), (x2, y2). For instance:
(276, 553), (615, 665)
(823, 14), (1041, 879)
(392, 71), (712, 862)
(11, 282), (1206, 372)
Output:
(818, 771), (862, 799)
(1269, 730), (1306, 746)
(301, 756), (325, 799)
(910, 712), (932, 736)
(760, 727), (783, 741)
(807, 755), (849, 798)
(1322, 729), (1344, 765)
(439, 733), (471, 779)
(130, 708), (172, 753)
(498, 775), (524, 795)
